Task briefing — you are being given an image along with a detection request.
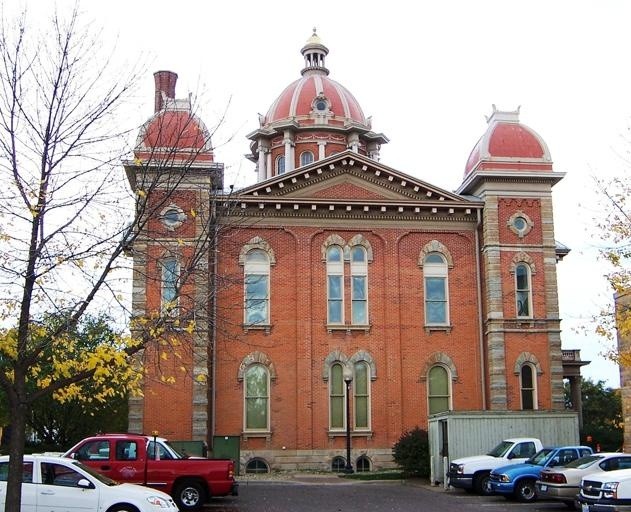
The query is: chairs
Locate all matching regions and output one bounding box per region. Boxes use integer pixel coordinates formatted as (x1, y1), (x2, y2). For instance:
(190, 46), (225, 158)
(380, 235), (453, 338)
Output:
(45, 463), (56, 483)
(605, 458), (619, 471)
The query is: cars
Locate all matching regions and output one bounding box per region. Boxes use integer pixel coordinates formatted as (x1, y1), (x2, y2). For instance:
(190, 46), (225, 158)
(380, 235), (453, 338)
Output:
(1, 456), (180, 512)
(447, 438), (631, 512)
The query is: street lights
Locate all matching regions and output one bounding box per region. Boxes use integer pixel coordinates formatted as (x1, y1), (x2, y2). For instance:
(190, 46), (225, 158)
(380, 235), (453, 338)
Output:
(342, 366), (354, 474)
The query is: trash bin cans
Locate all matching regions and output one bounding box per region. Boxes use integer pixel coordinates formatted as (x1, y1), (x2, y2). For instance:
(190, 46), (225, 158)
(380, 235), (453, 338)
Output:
(168, 435), (240, 476)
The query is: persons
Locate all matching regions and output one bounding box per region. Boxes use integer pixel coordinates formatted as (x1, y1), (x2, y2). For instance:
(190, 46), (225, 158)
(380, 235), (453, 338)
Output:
(581, 433), (601, 455)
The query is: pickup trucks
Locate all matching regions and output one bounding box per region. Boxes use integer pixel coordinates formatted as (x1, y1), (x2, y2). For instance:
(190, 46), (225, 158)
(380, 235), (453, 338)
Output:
(30, 434), (234, 511)
